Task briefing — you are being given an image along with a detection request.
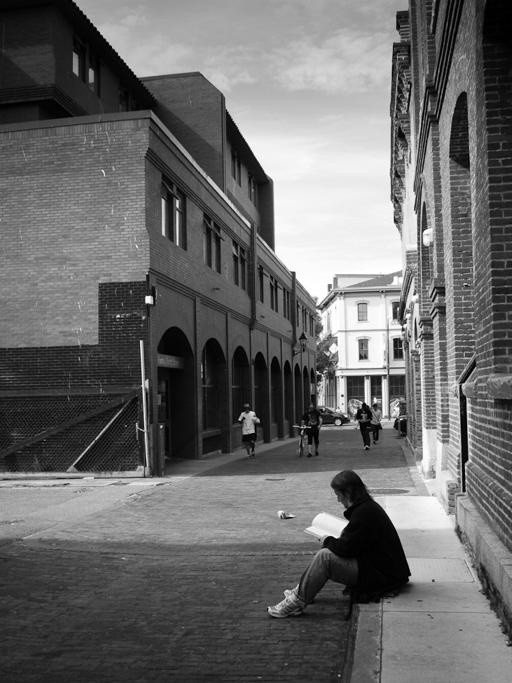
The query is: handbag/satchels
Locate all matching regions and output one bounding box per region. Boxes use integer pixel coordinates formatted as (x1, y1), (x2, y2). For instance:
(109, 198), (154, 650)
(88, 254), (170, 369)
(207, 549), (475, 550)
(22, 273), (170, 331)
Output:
(378, 423), (382, 430)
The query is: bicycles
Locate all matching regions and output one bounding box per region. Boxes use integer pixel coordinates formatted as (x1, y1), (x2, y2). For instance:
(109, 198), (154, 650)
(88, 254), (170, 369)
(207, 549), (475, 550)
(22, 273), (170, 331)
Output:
(291, 423), (313, 456)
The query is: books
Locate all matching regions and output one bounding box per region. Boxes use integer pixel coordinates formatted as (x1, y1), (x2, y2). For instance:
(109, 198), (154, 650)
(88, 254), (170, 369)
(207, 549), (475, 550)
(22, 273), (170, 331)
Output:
(303, 509), (350, 542)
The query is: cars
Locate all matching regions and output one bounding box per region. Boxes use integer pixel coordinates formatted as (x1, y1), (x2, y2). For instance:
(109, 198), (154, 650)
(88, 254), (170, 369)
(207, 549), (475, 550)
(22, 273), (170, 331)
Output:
(307, 405), (351, 427)
(392, 414), (408, 434)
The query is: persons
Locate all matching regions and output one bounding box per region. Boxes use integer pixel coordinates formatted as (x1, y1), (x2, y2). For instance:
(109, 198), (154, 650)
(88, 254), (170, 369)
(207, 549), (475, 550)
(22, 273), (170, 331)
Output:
(236, 402), (262, 459)
(355, 402), (374, 449)
(265, 469), (411, 618)
(369, 404), (383, 445)
(296, 401), (324, 458)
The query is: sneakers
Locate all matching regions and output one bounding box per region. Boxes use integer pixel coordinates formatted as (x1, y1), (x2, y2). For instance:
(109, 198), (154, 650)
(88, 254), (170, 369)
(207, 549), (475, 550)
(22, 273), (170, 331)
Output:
(266, 596), (303, 620)
(316, 452), (318, 456)
(373, 440), (376, 444)
(364, 445), (370, 450)
(307, 453), (312, 457)
(284, 583), (299, 597)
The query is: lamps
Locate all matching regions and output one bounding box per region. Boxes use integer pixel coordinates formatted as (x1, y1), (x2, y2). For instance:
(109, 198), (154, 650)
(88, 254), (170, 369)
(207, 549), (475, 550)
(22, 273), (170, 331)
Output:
(292, 331), (307, 356)
